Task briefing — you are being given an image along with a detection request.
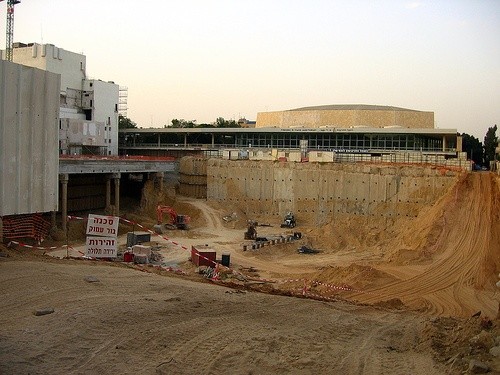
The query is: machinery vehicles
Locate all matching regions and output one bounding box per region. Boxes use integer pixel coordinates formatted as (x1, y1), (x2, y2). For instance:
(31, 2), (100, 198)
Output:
(153, 205), (191, 234)
(280, 212), (296, 229)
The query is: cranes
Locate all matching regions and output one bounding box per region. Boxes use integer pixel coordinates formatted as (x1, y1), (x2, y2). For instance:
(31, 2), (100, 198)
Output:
(0, 0), (22, 63)
